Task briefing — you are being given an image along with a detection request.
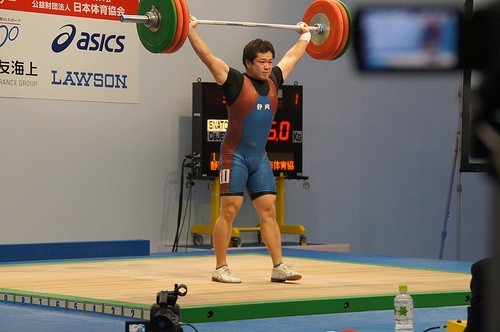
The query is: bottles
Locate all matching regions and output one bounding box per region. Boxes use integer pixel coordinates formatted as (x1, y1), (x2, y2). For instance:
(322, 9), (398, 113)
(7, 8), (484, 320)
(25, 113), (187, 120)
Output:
(394, 285), (414, 332)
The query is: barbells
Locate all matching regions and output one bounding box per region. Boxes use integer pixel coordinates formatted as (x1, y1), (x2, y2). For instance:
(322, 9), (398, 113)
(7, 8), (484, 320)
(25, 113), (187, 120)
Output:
(119, 0), (354, 61)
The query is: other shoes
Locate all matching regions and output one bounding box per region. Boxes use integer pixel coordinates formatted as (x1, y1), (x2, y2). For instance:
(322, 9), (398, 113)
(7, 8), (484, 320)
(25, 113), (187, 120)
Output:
(212, 265), (242, 284)
(270, 264), (301, 282)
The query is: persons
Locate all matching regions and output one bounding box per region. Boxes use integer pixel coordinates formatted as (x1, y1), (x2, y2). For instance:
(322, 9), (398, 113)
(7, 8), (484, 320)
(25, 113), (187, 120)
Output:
(188, 15), (312, 283)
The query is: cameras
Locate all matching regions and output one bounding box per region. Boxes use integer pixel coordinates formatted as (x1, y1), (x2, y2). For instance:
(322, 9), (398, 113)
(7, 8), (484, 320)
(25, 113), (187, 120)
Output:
(354, 5), (500, 159)
